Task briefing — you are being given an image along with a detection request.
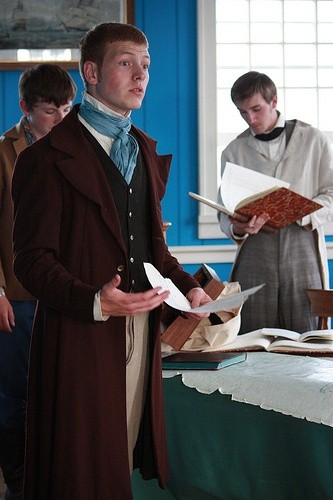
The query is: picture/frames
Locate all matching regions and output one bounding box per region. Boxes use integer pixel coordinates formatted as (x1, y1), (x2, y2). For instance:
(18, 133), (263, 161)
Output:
(0, 0), (136, 70)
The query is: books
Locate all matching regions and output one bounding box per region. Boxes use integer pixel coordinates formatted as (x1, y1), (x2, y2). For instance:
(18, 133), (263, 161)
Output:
(161, 162), (333, 370)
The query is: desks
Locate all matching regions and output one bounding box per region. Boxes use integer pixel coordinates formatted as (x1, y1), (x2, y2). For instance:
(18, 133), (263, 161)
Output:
(132, 349), (333, 500)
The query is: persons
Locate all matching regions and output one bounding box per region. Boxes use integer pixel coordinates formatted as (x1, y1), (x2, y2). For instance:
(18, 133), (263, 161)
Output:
(0, 63), (77, 500)
(12, 23), (212, 500)
(216, 71), (333, 334)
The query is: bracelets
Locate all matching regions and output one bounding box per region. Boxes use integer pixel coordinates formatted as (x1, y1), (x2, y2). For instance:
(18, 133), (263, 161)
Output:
(0, 287), (5, 297)
